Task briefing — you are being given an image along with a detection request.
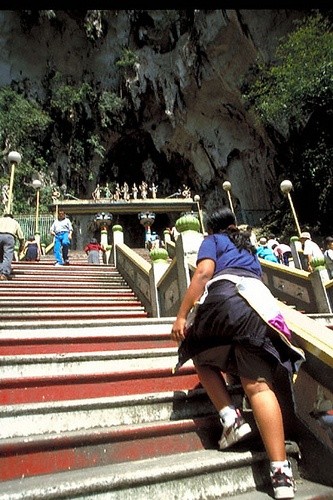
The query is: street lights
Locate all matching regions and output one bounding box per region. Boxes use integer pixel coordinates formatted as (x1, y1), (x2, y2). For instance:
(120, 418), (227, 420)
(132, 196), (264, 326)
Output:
(222, 180), (239, 227)
(194, 194), (205, 234)
(6, 151), (22, 214)
(32, 180), (42, 260)
(280, 180), (302, 236)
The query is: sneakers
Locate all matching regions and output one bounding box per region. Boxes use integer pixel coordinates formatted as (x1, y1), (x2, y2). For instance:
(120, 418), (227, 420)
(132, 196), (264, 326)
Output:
(217, 408), (252, 450)
(270, 461), (297, 498)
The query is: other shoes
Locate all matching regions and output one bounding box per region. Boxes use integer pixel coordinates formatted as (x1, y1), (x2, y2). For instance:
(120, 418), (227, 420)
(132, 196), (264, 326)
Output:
(1, 274), (8, 281)
(65, 259), (70, 263)
(54, 263), (63, 267)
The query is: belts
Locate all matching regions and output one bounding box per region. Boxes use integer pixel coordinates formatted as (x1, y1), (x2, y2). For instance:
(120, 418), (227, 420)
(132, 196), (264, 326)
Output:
(0, 232), (16, 238)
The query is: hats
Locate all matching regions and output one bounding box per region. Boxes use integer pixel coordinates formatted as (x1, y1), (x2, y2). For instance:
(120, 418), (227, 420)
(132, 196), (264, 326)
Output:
(299, 232), (312, 240)
(258, 238), (269, 246)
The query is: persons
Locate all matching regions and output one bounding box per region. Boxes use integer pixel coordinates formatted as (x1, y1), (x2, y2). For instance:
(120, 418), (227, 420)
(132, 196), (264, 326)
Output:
(50, 209), (73, 266)
(93, 181), (158, 200)
(22, 235), (40, 261)
(0, 213), (25, 281)
(323, 236), (333, 278)
(85, 238), (106, 264)
(163, 225), (179, 243)
(170, 206), (298, 500)
(299, 231), (323, 272)
(52, 188), (60, 200)
(148, 232), (160, 249)
(247, 226), (292, 264)
(182, 186), (191, 198)
